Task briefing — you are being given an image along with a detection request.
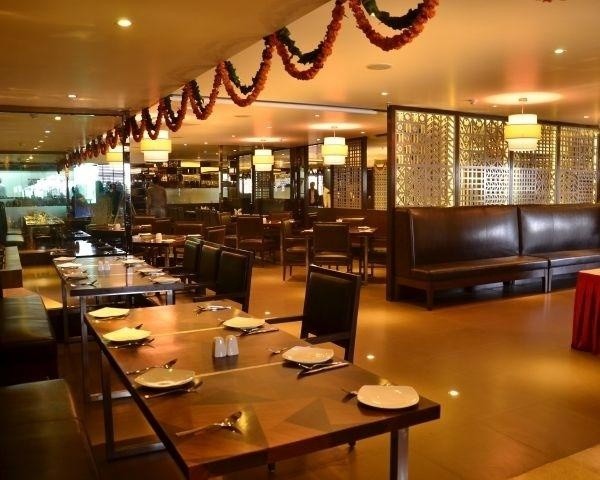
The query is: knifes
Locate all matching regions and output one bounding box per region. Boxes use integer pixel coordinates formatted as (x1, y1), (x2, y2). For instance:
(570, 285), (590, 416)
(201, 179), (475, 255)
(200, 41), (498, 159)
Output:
(198, 308), (233, 312)
(297, 362), (352, 375)
(236, 329), (279, 336)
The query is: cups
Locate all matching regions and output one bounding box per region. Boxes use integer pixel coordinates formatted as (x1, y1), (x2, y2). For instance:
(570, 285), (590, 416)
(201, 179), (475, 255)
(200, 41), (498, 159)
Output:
(224, 335), (239, 357)
(104, 261), (110, 270)
(155, 233), (162, 241)
(211, 336), (227, 358)
(97, 260), (103, 271)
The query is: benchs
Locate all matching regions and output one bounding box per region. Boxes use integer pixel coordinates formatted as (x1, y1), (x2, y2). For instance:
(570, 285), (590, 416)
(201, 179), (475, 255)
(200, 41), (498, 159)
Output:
(1, 246), (23, 287)
(1, 293), (59, 386)
(0, 199), (25, 243)
(0, 376), (100, 480)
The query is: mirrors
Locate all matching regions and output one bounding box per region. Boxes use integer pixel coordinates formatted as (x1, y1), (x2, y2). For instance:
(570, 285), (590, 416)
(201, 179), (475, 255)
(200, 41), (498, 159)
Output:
(0, 109), (128, 259)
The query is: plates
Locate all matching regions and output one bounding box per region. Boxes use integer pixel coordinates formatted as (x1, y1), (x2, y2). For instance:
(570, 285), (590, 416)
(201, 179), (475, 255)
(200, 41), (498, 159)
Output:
(222, 316), (265, 330)
(58, 263), (82, 268)
(281, 345), (335, 364)
(103, 327), (152, 343)
(53, 256), (76, 262)
(152, 276), (181, 283)
(136, 267), (163, 275)
(357, 384), (419, 410)
(64, 273), (90, 279)
(133, 367), (195, 390)
(87, 306), (131, 318)
(122, 259), (145, 264)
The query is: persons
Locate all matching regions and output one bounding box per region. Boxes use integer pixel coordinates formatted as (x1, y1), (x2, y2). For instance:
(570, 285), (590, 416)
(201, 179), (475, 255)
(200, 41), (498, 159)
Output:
(71, 187), (88, 217)
(105, 184), (121, 216)
(308, 182), (319, 206)
(147, 178), (167, 219)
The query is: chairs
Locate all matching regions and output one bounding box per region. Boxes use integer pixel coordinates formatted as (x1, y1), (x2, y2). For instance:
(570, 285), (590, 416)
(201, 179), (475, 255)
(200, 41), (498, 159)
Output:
(268, 210), (293, 220)
(358, 236), (388, 279)
(151, 215), (174, 236)
(204, 225), (227, 245)
(328, 217), (368, 273)
(145, 238), (221, 307)
(194, 208), (220, 226)
(168, 206), (185, 219)
(130, 214), (157, 236)
(172, 220), (205, 259)
(217, 211), (236, 241)
(394, 204), (549, 311)
(280, 216), (312, 282)
(140, 235), (203, 298)
(174, 245), (256, 314)
(510, 202), (600, 293)
(312, 221), (353, 273)
(235, 214), (276, 268)
(260, 262), (363, 472)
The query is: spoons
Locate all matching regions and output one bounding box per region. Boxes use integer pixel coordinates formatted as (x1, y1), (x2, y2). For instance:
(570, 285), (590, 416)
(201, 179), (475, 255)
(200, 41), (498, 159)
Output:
(126, 358), (178, 377)
(73, 280), (97, 286)
(175, 411), (243, 437)
(299, 361), (348, 369)
(196, 305), (234, 310)
(145, 379), (205, 400)
(108, 338), (158, 349)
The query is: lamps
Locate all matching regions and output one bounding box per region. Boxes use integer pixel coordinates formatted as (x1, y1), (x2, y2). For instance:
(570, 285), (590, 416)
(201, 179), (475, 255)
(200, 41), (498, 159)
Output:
(503, 98), (542, 153)
(139, 116), (172, 166)
(320, 127), (348, 166)
(252, 140), (275, 172)
(129, 129), (145, 165)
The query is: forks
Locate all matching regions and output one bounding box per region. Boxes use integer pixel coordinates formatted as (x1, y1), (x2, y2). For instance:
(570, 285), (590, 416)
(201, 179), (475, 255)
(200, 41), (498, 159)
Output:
(267, 342), (310, 354)
(341, 383), (391, 396)
